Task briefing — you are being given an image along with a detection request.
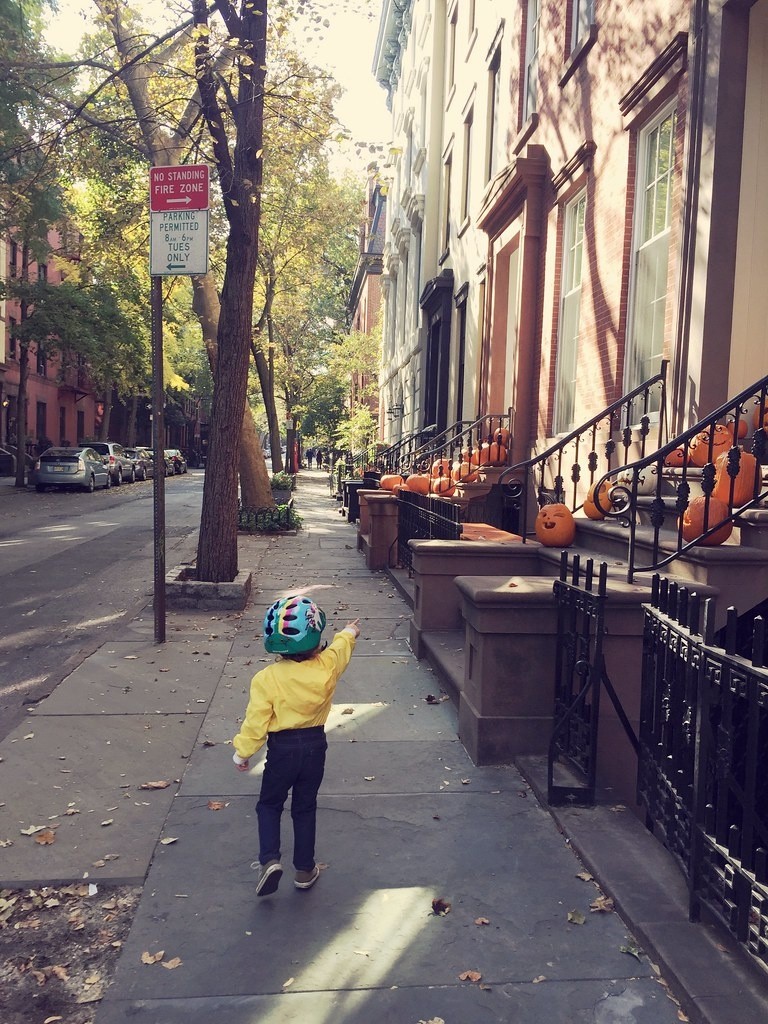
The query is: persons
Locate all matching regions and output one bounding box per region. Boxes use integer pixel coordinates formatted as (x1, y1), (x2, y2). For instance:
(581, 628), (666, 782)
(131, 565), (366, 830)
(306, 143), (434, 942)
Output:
(231, 596), (362, 899)
(306, 449), (314, 470)
(317, 450), (322, 469)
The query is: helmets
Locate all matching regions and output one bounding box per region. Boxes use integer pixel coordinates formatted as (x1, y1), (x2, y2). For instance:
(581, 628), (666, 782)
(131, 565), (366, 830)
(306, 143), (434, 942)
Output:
(262, 596), (327, 658)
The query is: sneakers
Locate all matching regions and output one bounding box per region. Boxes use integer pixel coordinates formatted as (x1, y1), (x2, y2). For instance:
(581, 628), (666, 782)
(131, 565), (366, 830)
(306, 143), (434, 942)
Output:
(255, 860), (282, 897)
(294, 865), (318, 889)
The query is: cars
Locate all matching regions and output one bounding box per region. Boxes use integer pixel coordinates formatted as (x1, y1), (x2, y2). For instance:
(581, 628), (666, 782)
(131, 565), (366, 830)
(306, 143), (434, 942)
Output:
(142, 447), (175, 478)
(165, 450), (188, 475)
(35, 447), (111, 493)
(123, 447), (155, 481)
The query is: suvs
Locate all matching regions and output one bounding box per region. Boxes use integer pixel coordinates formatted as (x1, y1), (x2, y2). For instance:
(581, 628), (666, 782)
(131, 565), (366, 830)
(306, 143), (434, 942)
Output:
(79, 442), (136, 485)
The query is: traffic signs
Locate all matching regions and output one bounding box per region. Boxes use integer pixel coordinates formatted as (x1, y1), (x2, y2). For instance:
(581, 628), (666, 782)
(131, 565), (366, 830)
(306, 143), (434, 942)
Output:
(149, 165), (210, 212)
(149, 211), (209, 276)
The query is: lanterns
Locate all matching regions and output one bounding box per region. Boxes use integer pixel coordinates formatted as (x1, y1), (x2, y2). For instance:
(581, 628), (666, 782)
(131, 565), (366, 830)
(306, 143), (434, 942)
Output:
(753, 398), (768, 433)
(616, 466), (658, 495)
(711, 444), (763, 508)
(534, 493), (576, 547)
(676, 493), (733, 547)
(583, 480), (615, 519)
